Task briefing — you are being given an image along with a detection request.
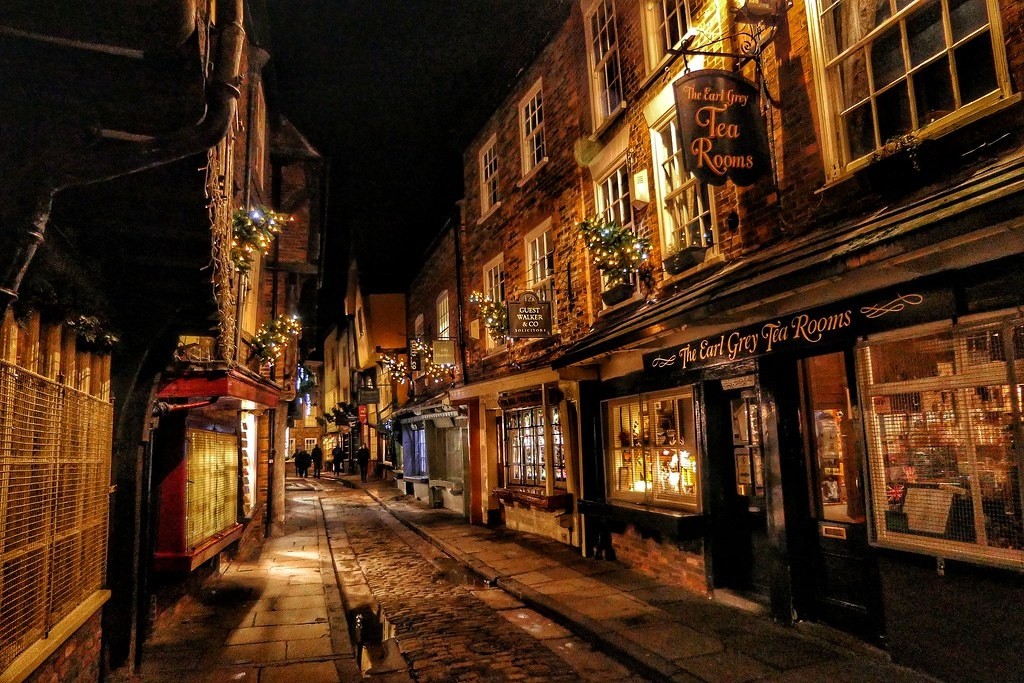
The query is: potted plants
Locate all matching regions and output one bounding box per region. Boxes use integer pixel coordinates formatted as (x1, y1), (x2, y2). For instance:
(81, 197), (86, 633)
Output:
(662, 237), (707, 276)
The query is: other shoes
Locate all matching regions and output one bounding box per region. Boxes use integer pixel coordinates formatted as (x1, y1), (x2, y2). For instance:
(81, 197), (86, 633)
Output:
(361, 480), (367, 484)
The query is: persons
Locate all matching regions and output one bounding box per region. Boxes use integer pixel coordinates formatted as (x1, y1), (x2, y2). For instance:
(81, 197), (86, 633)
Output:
(292, 448), (311, 478)
(356, 443), (371, 483)
(330, 443), (344, 476)
(310, 444), (323, 478)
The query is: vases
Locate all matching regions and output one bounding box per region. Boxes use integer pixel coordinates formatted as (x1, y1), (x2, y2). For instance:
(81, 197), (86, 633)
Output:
(600, 283), (635, 306)
